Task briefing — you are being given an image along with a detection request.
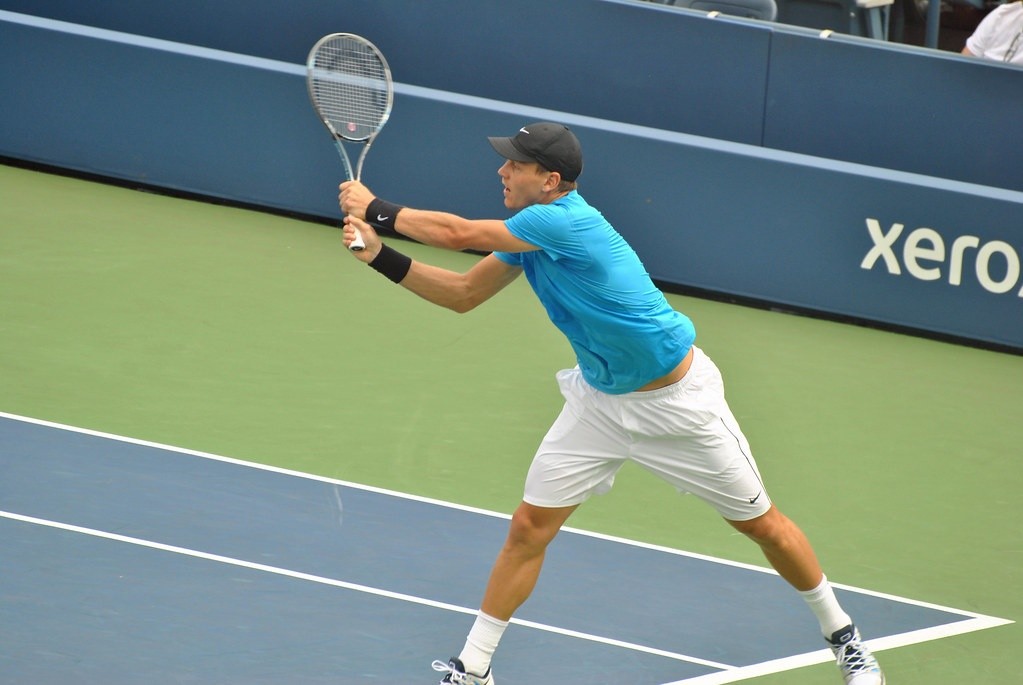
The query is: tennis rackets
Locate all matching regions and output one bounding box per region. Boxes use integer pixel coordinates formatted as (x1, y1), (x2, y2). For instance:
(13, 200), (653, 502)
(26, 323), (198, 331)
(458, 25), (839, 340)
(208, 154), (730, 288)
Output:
(305, 32), (395, 254)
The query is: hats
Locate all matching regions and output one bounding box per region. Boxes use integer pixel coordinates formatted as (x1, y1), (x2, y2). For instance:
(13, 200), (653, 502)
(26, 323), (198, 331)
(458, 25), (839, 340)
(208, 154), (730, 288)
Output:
(487, 122), (582, 183)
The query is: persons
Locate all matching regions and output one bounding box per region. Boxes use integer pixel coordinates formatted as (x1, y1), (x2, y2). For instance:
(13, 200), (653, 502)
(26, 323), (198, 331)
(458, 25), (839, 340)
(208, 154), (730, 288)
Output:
(961, 0), (1023, 67)
(338, 123), (887, 685)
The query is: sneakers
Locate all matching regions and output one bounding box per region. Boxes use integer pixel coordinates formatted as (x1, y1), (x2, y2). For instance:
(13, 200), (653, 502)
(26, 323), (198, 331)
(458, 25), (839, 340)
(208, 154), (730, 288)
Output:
(826, 621), (886, 685)
(432, 656), (494, 685)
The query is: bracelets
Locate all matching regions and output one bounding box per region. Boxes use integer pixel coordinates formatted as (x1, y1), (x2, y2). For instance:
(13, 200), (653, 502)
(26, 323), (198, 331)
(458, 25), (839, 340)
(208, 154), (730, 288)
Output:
(365, 198), (405, 232)
(367, 242), (412, 284)
